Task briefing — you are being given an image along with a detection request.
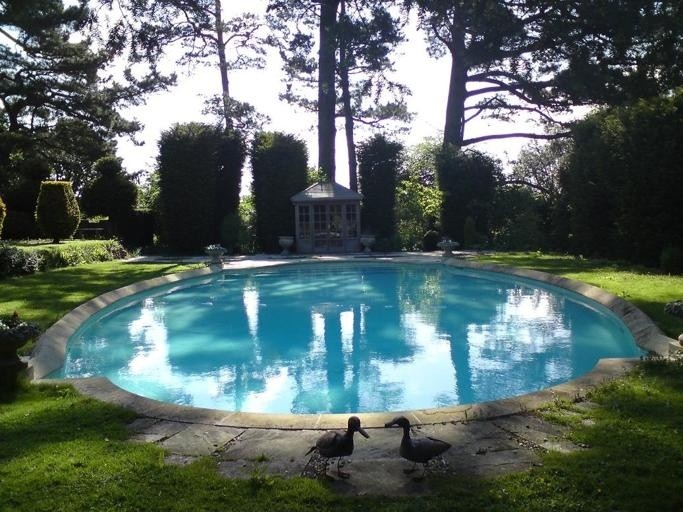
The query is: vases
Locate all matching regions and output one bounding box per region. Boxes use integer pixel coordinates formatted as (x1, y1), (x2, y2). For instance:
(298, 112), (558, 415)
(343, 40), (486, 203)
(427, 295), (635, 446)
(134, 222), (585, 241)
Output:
(278, 236), (295, 256)
(360, 235), (376, 252)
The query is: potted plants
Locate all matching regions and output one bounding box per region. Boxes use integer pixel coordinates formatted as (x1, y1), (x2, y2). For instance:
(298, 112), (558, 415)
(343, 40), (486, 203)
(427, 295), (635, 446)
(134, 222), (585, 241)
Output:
(204, 244), (228, 263)
(436, 240), (460, 256)
(664, 300), (683, 345)
(0, 310), (43, 373)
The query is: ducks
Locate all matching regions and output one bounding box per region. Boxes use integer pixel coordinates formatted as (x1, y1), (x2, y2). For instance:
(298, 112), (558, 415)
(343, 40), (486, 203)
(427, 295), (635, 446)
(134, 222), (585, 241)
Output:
(316, 415), (371, 482)
(383, 416), (453, 481)
